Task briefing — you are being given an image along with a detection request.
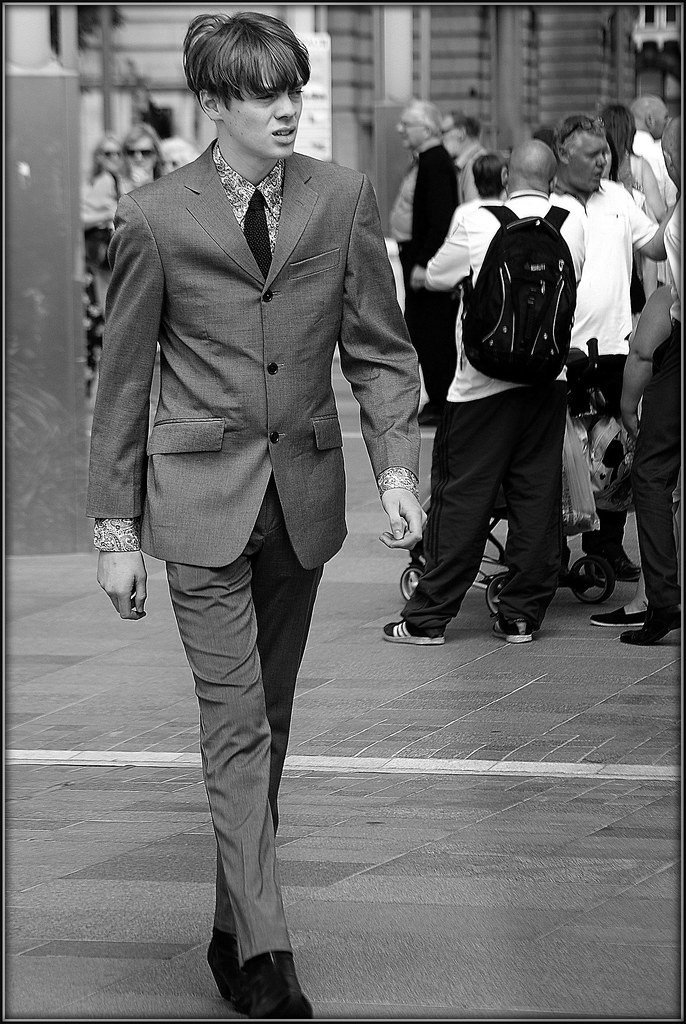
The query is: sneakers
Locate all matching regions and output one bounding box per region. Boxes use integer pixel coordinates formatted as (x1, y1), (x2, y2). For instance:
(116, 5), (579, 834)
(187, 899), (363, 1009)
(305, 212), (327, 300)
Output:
(490, 613), (532, 643)
(600, 545), (641, 581)
(383, 619), (444, 644)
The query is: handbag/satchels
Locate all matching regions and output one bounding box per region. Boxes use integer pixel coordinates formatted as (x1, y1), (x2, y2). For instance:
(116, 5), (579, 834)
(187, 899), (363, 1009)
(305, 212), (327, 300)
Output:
(83, 170), (121, 270)
(561, 404), (600, 537)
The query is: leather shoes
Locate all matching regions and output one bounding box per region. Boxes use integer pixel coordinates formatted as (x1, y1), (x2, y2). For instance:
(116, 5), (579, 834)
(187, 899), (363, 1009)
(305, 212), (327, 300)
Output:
(620, 604), (681, 645)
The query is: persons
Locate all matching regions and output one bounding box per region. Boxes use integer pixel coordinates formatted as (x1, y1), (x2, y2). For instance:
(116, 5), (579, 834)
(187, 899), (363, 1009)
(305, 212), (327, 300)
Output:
(77, 97), (681, 652)
(90, 11), (427, 1018)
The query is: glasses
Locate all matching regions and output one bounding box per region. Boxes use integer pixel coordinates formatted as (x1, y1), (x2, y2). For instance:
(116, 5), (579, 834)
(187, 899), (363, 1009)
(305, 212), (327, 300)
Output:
(560, 115), (604, 143)
(127, 149), (155, 158)
(100, 151), (119, 158)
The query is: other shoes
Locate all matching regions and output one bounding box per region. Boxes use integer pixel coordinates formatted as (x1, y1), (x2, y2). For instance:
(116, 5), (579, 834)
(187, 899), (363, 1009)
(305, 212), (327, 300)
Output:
(417, 410), (437, 426)
(207, 929), (242, 1003)
(244, 951), (312, 1020)
(587, 607), (647, 627)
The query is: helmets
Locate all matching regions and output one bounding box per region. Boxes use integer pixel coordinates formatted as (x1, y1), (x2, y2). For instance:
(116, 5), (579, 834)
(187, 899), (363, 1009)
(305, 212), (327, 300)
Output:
(584, 416), (634, 511)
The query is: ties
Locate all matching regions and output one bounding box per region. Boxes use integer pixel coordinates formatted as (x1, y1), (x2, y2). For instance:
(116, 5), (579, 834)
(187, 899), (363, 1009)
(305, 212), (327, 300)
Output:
(244, 189), (272, 281)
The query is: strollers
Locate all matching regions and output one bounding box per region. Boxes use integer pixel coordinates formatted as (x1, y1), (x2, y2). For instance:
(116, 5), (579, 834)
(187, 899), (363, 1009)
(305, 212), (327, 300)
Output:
(397, 336), (617, 622)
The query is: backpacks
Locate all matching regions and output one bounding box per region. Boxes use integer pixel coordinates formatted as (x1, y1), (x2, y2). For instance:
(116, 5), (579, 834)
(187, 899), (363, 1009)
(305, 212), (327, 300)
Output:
(462, 205), (577, 385)
